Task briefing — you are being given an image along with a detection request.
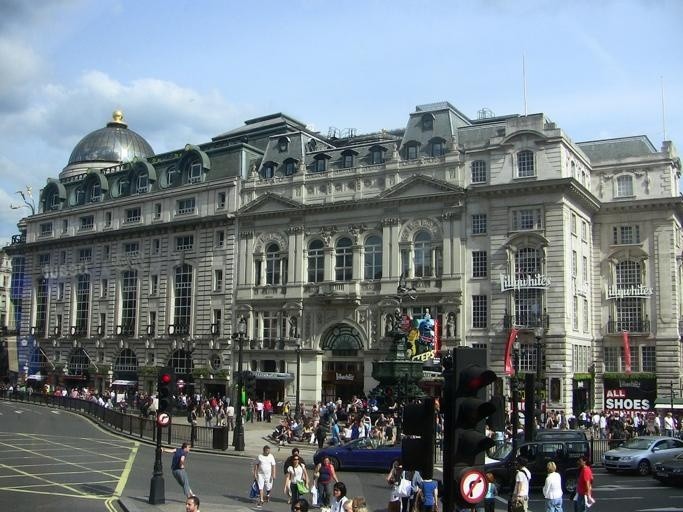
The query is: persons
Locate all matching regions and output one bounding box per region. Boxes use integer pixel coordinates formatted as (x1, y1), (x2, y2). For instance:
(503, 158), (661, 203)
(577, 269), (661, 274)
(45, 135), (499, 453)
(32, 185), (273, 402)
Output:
(574, 456), (594, 512)
(544, 409), (683, 439)
(542, 461), (563, 512)
(485, 473), (498, 512)
(268, 391), (443, 449)
(1, 384), (130, 415)
(131, 388), (273, 431)
(283, 448), (439, 512)
(186, 496), (200, 512)
(254, 445), (276, 508)
(161, 442), (195, 497)
(512, 456), (531, 512)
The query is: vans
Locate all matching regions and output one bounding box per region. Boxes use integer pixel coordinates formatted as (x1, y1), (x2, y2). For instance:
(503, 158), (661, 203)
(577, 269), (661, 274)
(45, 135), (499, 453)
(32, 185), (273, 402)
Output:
(509, 428), (589, 460)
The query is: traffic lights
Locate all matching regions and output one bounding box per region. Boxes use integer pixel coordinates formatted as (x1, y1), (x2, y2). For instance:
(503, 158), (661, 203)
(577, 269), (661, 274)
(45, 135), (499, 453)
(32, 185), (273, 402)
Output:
(401, 397), (434, 481)
(524, 372), (543, 435)
(448, 345), (500, 468)
(157, 367), (173, 411)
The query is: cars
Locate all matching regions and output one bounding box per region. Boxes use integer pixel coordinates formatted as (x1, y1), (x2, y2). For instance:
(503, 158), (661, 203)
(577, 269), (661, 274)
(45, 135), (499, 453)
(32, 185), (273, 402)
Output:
(649, 448), (682, 490)
(599, 433), (682, 479)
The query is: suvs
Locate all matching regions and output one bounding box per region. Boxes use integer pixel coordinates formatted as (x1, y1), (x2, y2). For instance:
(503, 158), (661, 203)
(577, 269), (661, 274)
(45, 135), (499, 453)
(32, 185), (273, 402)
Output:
(480, 437), (589, 497)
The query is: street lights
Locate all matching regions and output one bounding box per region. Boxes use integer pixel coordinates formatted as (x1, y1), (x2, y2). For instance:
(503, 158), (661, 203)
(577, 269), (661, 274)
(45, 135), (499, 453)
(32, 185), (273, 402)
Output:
(179, 334), (196, 396)
(293, 335), (305, 418)
(669, 390), (678, 412)
(533, 325), (544, 426)
(232, 313), (248, 450)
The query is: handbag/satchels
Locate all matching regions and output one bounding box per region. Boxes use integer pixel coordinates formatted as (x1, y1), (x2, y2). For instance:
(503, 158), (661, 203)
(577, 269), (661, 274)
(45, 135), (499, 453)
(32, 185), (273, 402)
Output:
(393, 478), (411, 498)
(310, 485), (318, 504)
(507, 496), (526, 512)
(297, 480), (309, 495)
(249, 480), (259, 498)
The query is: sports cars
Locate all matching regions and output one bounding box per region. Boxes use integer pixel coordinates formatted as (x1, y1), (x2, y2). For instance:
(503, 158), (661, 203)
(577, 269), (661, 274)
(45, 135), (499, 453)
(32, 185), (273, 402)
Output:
(313, 436), (403, 474)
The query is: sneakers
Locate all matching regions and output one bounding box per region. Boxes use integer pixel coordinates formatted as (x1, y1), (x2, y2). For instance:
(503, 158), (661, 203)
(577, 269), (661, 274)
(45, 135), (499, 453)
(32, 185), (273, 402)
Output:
(256, 497), (271, 508)
